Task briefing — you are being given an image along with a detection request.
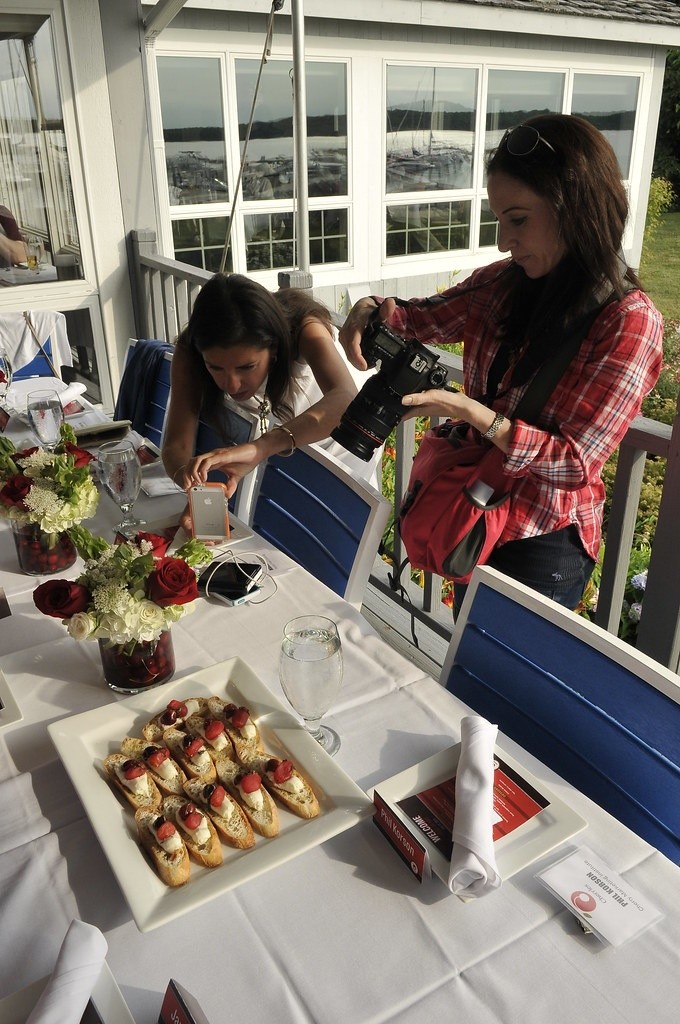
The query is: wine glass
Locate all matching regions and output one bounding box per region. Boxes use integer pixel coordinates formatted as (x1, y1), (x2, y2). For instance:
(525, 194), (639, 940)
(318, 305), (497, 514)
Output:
(97, 440), (147, 536)
(27, 388), (65, 453)
(278, 614), (343, 757)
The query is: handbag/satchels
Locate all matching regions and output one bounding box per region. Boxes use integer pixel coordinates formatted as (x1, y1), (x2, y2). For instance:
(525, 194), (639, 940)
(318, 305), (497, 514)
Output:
(398, 419), (518, 585)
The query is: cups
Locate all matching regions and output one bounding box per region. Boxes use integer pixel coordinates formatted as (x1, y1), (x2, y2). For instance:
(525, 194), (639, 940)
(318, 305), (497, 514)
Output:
(22, 233), (44, 275)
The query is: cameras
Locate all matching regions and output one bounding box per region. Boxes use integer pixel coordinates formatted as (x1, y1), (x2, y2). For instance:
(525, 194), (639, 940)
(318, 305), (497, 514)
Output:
(329, 323), (447, 463)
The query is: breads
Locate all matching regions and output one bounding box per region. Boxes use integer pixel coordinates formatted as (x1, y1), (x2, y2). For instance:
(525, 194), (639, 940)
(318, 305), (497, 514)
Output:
(102, 696), (320, 886)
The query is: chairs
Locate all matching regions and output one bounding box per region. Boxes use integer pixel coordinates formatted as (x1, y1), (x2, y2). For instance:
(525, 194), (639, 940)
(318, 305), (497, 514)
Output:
(0, 308), (679, 867)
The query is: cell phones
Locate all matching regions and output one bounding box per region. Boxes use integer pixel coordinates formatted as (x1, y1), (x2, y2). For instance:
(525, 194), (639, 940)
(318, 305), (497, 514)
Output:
(188, 482), (230, 541)
(208, 584), (261, 606)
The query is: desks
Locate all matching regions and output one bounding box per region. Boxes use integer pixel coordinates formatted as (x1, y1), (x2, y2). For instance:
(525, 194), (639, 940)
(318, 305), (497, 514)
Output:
(0, 378), (679, 1024)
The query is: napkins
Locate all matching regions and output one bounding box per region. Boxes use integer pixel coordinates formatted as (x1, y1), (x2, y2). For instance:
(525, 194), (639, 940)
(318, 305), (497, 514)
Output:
(22, 382), (87, 418)
(114, 431), (144, 451)
(446, 715), (503, 899)
(25, 918), (108, 1024)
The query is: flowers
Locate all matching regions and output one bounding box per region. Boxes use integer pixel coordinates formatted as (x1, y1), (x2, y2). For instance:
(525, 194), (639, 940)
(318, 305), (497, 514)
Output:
(31, 524), (214, 657)
(0, 422), (100, 551)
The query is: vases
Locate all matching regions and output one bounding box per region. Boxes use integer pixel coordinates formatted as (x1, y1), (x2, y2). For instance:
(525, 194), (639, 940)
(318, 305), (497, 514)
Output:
(6, 517), (78, 577)
(96, 630), (176, 696)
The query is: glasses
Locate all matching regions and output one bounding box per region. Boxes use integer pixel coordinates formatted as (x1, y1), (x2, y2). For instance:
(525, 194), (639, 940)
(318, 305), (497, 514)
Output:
(498, 124), (557, 158)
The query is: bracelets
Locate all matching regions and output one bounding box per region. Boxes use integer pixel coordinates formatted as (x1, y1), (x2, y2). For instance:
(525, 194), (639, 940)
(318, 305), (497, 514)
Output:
(272, 427), (296, 457)
(173, 465), (188, 493)
(481, 412), (504, 439)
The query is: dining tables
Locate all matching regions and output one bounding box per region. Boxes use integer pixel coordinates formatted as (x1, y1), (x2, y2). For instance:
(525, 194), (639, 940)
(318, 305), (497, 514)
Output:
(0, 260), (90, 369)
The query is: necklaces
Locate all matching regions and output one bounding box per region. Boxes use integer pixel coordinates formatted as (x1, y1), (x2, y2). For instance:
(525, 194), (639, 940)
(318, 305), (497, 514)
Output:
(253, 376), (270, 434)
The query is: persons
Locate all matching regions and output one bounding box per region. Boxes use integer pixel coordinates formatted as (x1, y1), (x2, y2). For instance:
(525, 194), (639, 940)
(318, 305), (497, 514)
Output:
(162, 274), (359, 546)
(0, 205), (27, 267)
(339, 114), (664, 624)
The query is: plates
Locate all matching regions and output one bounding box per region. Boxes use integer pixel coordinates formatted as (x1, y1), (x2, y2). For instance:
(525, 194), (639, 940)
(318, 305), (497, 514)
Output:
(47, 656), (377, 934)
